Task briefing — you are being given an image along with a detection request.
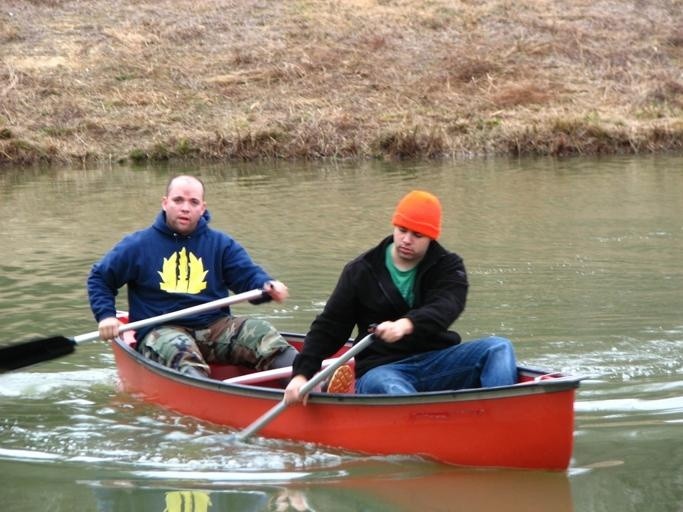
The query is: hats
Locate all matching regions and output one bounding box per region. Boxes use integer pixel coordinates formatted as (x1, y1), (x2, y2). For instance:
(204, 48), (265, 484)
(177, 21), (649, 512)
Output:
(391, 189), (442, 241)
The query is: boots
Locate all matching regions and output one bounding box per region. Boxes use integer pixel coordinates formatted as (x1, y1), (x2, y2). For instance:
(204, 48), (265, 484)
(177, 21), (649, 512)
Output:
(265, 346), (354, 394)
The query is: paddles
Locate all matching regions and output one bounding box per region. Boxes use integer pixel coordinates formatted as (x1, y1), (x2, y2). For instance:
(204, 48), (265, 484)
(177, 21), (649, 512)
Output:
(1, 282), (273, 374)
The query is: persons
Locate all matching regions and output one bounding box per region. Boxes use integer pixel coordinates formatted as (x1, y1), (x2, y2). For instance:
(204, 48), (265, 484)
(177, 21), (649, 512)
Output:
(284, 190), (517, 407)
(87, 175), (353, 395)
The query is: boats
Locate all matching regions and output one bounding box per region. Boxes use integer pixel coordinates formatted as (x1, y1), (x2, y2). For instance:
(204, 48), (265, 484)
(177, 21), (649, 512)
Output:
(110, 308), (593, 475)
